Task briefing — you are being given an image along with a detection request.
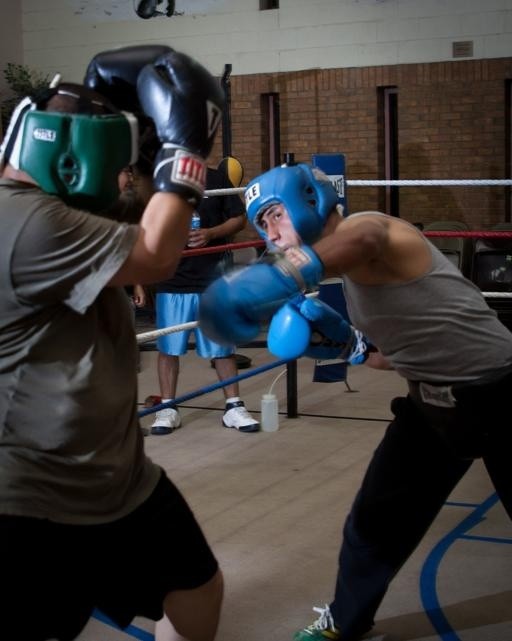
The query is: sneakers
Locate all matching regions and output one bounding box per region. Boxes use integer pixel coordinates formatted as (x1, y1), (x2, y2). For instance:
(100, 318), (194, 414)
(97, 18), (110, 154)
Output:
(292, 603), (372, 641)
(151, 398), (181, 436)
(222, 396), (260, 432)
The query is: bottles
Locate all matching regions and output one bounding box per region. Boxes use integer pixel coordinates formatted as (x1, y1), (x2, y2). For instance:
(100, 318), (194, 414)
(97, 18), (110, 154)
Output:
(190, 210), (201, 231)
(261, 394), (279, 434)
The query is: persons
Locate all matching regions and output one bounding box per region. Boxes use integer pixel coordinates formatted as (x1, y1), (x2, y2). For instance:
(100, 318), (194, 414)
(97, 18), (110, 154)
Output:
(199, 163), (512, 641)
(0, 44), (224, 641)
(150, 169), (260, 432)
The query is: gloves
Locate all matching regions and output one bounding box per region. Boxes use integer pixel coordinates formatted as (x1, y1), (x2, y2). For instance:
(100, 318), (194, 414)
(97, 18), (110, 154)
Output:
(85, 43), (175, 175)
(135, 50), (227, 209)
(198, 247), (325, 347)
(267, 297), (368, 364)
